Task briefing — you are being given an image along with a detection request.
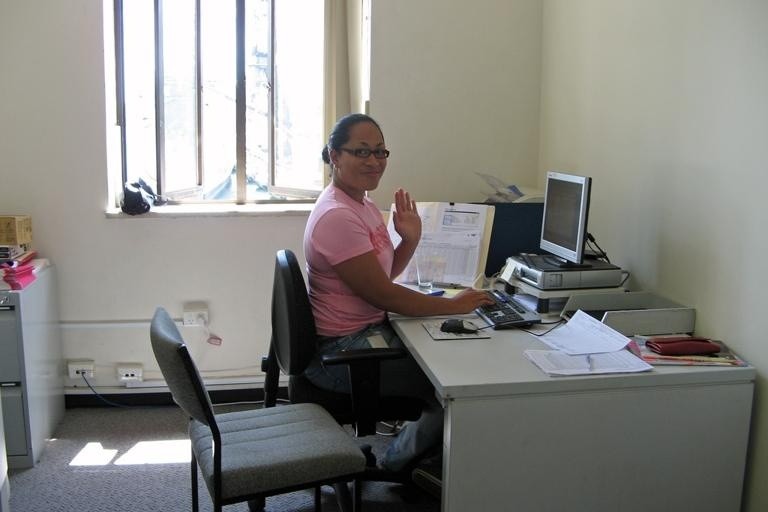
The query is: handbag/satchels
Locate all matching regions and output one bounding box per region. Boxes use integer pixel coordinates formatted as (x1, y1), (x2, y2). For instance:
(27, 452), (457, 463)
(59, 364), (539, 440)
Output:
(119, 177), (154, 215)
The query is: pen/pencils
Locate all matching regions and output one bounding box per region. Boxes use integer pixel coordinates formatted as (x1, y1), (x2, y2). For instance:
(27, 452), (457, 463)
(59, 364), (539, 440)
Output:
(428, 291), (445, 296)
(586, 354), (592, 370)
(493, 293), (506, 303)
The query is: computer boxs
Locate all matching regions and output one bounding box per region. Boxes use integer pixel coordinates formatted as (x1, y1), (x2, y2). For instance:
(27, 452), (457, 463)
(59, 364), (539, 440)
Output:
(506, 254), (622, 290)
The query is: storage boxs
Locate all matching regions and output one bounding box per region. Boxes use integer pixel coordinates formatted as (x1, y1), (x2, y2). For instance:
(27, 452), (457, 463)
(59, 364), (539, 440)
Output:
(0, 214), (35, 247)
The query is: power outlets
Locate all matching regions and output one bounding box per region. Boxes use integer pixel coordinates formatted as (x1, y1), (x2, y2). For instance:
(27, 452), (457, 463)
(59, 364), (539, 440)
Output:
(116, 364), (144, 382)
(183, 309), (208, 327)
(66, 359), (95, 380)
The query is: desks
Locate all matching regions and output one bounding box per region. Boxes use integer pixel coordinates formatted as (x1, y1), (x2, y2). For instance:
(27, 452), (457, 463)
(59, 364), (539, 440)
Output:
(387, 282), (757, 511)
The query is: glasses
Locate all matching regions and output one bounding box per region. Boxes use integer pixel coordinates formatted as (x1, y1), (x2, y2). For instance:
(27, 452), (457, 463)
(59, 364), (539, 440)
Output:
(336, 146), (390, 159)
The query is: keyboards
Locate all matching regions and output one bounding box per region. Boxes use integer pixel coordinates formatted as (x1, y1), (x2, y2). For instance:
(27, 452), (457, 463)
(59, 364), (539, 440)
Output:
(475, 289), (542, 330)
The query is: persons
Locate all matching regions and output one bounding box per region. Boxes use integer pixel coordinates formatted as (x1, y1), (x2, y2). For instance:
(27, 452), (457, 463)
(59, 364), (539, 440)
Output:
(302, 114), (496, 502)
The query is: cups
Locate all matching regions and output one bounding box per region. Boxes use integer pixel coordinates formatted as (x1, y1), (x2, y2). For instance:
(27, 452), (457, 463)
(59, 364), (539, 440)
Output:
(414, 250), (438, 291)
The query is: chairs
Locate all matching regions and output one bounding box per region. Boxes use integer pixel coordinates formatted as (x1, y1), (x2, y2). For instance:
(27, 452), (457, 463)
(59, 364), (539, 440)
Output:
(262, 249), (406, 437)
(151, 306), (367, 512)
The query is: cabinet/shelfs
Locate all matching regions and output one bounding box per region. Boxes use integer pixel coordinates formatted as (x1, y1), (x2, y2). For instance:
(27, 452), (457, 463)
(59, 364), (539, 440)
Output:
(0, 263), (68, 471)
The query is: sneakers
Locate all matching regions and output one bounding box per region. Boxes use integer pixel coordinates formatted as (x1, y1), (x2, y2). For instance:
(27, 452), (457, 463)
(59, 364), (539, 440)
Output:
(412, 461), (442, 492)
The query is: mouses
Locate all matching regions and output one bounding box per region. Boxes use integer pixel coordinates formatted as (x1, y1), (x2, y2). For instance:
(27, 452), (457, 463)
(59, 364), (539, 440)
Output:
(440, 319), (478, 333)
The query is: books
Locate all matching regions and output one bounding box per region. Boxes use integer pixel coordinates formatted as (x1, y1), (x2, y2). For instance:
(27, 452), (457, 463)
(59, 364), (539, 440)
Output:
(0, 243), (50, 292)
(628, 335), (750, 370)
(383, 197), (497, 290)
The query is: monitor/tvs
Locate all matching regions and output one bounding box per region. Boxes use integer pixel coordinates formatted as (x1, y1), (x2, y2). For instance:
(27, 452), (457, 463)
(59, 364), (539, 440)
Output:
(539, 171), (593, 268)
